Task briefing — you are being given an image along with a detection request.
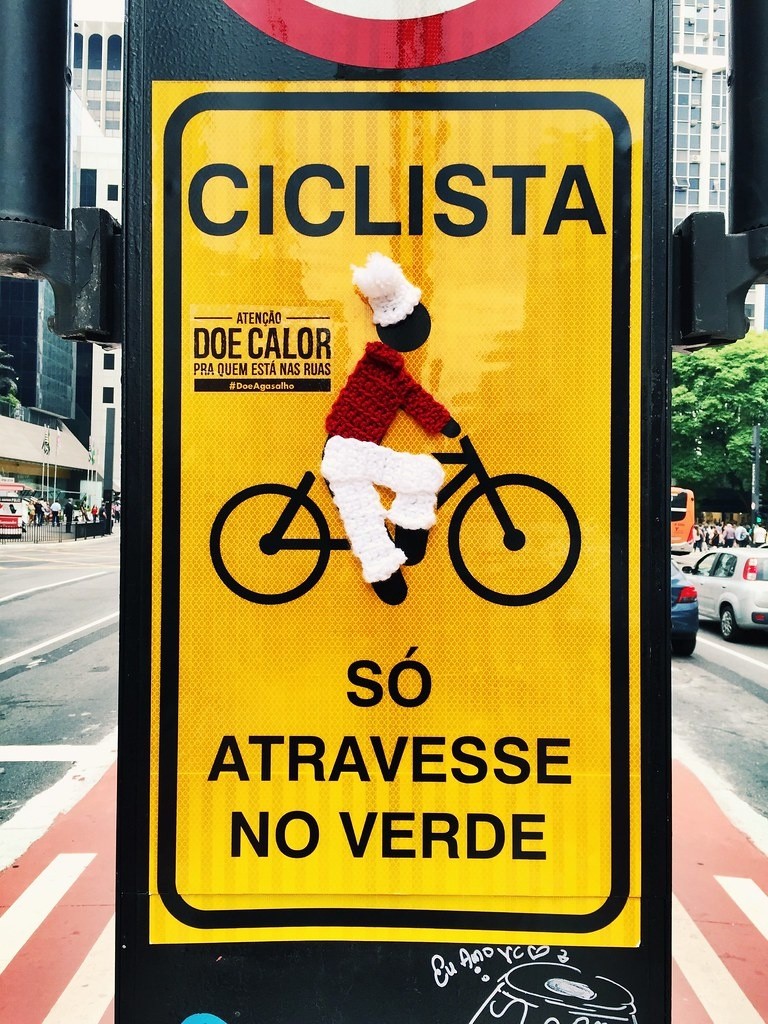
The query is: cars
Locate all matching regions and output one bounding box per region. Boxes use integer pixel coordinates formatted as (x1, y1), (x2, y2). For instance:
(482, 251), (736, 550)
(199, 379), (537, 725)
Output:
(670, 557), (699, 659)
(680, 548), (768, 642)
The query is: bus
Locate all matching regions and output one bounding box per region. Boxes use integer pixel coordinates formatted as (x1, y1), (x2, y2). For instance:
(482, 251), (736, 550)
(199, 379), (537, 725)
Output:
(670, 485), (695, 551)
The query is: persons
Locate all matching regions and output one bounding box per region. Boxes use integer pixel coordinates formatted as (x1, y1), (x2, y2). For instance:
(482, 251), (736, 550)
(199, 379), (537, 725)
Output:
(318, 254), (461, 608)
(28, 496), (121, 535)
(694, 520), (768, 555)
